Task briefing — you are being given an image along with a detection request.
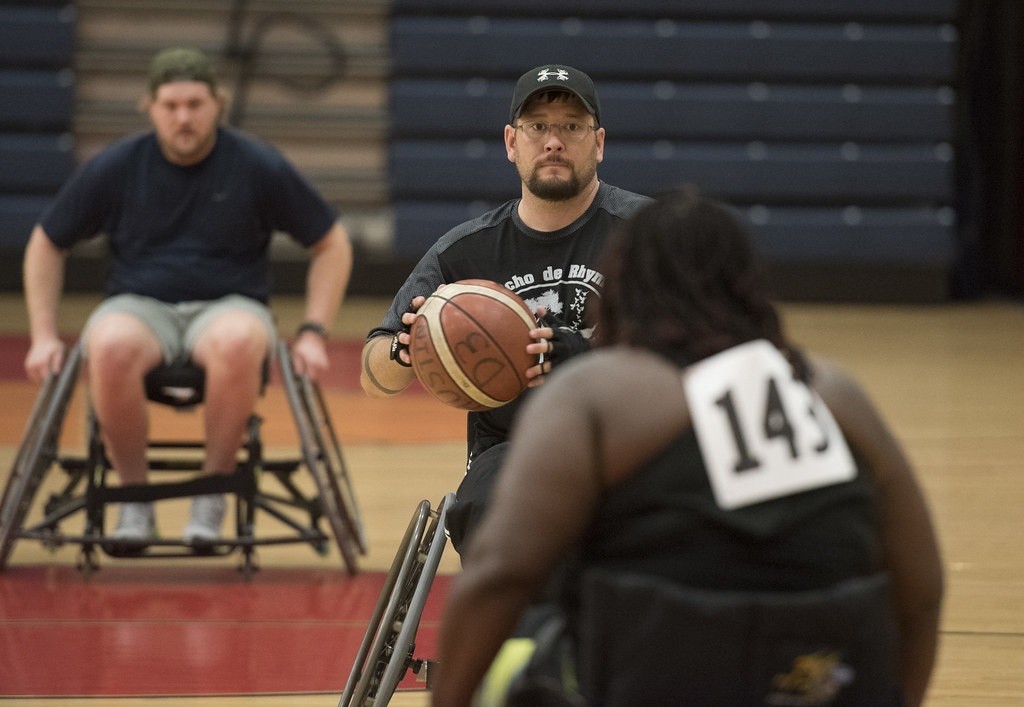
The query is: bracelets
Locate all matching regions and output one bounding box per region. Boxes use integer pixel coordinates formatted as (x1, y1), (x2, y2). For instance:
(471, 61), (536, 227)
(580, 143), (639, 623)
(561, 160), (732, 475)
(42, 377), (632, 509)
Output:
(297, 320), (332, 341)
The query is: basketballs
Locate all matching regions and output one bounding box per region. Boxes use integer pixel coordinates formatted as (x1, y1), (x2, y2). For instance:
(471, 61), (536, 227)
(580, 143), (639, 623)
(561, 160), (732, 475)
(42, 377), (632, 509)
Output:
(407, 276), (545, 414)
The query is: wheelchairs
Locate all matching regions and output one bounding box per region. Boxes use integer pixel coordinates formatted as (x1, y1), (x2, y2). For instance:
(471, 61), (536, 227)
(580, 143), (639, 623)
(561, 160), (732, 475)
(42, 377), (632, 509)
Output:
(1, 316), (367, 582)
(335, 491), (465, 706)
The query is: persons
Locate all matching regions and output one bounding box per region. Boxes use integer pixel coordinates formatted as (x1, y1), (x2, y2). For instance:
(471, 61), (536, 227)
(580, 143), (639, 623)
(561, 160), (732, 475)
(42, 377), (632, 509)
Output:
(360, 65), (945, 707)
(24, 50), (353, 557)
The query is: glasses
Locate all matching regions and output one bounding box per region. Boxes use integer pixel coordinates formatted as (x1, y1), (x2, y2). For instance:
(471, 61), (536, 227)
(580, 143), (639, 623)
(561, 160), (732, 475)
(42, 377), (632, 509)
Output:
(515, 120), (599, 137)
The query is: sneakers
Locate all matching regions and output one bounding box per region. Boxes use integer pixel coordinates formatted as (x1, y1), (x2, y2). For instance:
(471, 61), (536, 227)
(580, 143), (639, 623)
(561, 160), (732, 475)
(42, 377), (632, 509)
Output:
(112, 504), (158, 540)
(185, 494), (227, 543)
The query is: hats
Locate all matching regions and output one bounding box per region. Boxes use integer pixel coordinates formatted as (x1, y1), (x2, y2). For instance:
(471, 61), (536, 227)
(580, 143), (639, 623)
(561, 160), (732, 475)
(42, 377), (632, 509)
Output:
(510, 64), (602, 125)
(146, 48), (217, 99)
